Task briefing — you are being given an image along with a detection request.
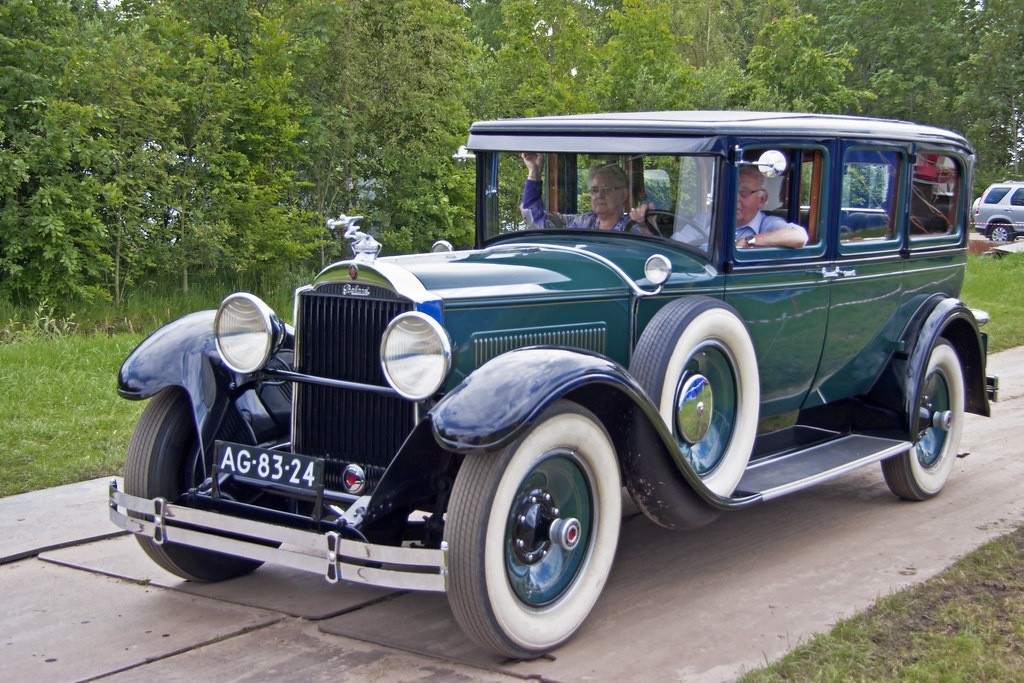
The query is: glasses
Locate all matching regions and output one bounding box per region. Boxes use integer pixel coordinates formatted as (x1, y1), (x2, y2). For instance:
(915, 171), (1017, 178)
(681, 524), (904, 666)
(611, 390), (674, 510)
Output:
(738, 187), (766, 198)
(587, 186), (627, 196)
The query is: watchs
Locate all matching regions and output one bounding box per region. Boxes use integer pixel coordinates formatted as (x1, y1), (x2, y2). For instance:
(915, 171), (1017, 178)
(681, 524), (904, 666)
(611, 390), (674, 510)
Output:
(745, 235), (755, 248)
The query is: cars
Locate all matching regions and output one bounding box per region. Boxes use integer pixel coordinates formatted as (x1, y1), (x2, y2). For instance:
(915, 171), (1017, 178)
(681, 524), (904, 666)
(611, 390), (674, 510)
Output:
(106, 110), (1000, 664)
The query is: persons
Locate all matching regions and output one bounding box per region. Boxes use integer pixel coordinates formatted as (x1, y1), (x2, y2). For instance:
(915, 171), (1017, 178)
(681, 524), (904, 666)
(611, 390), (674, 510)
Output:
(630, 164), (809, 252)
(519, 152), (641, 234)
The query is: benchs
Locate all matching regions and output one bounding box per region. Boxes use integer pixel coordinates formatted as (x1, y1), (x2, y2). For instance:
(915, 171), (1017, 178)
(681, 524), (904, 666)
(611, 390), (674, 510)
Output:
(762, 208), (947, 242)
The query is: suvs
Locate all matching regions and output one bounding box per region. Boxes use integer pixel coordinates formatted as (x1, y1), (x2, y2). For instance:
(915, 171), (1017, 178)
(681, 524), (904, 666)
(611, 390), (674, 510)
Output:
(970, 180), (1024, 242)
